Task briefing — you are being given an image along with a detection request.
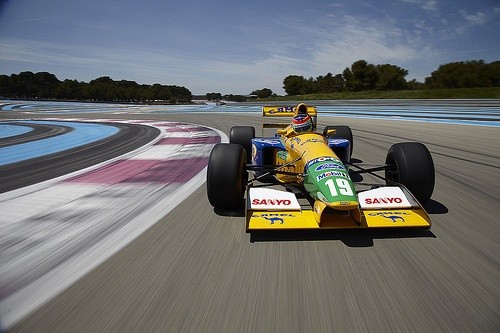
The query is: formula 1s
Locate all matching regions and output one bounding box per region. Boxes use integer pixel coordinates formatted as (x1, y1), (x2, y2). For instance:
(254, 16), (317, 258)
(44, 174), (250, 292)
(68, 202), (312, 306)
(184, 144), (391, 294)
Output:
(207, 102), (436, 234)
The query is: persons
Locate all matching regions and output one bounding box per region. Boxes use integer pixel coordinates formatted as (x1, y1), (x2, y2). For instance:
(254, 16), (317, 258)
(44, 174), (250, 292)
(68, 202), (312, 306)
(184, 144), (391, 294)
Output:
(290, 113), (313, 134)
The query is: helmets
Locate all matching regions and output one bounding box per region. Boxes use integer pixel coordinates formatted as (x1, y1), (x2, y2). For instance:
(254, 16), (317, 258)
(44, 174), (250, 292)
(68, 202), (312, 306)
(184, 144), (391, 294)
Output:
(292, 114), (312, 134)
